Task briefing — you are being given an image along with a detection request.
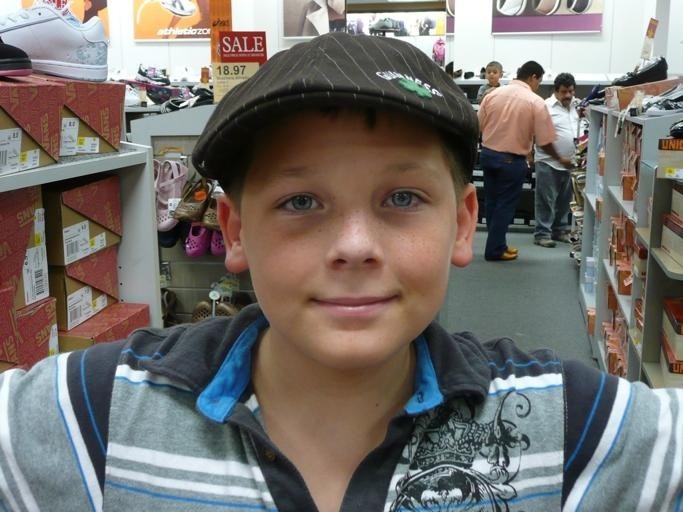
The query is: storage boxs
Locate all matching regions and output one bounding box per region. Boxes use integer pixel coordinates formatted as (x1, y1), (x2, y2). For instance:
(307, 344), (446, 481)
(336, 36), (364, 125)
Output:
(655, 136), (681, 382)
(0, 68), (151, 377)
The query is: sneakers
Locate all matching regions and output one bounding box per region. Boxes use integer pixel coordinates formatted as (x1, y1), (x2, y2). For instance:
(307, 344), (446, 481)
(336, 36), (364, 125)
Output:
(641, 82), (683, 116)
(124, 84), (139, 107)
(134, 63), (170, 86)
(0, 4), (108, 83)
(501, 246), (518, 260)
(553, 233), (578, 244)
(534, 236), (558, 248)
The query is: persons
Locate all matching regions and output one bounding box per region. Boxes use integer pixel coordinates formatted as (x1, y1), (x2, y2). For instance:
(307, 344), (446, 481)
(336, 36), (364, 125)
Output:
(1, 33), (683, 511)
(476, 61), (575, 261)
(476, 62), (505, 104)
(533, 72), (584, 247)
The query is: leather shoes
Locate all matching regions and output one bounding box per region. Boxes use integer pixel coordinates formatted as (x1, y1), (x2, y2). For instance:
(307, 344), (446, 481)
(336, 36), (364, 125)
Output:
(612, 55), (668, 87)
(452, 67), (486, 78)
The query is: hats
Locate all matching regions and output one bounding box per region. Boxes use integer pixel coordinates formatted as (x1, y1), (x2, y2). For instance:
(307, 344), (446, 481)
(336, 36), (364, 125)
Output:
(191, 32), (477, 183)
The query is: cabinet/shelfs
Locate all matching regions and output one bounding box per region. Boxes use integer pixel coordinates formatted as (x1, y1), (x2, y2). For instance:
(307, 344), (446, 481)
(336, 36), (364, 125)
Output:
(577, 102), (681, 383)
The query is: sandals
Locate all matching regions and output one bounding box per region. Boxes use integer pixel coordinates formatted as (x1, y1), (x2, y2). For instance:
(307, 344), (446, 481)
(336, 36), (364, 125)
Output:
(160, 89), (213, 114)
(153, 159), (225, 257)
(191, 293), (252, 323)
